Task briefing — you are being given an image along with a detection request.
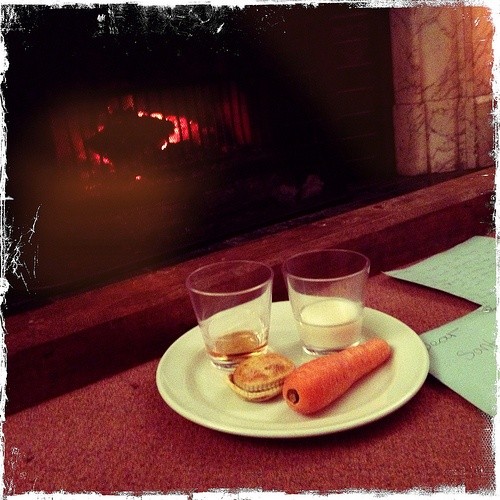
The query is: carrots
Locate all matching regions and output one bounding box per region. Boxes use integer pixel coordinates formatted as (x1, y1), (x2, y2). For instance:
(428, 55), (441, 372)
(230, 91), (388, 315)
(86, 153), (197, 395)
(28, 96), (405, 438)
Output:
(282, 337), (390, 416)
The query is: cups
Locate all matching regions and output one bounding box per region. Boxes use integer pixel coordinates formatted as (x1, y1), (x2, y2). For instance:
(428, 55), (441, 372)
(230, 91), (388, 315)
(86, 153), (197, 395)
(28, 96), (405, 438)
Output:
(280, 249), (369, 356)
(185, 260), (274, 369)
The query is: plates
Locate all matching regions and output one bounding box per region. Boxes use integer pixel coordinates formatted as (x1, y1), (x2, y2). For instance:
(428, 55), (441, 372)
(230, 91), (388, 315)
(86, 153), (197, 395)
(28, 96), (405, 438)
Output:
(156, 301), (431, 439)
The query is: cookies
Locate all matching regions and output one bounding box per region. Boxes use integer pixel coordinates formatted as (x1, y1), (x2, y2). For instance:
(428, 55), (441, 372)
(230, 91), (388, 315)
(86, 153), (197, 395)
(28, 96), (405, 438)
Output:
(226, 352), (296, 403)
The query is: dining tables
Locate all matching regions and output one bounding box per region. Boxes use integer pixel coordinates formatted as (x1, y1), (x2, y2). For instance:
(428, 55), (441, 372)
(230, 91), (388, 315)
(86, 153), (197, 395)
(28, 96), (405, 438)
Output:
(4, 166), (496, 491)
